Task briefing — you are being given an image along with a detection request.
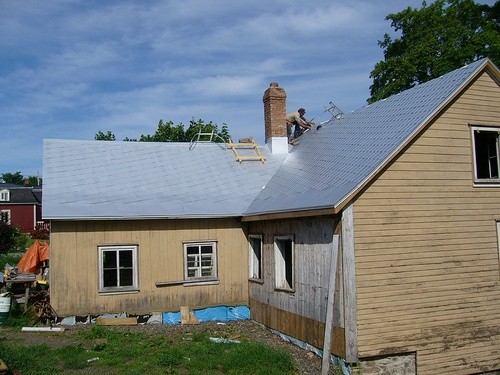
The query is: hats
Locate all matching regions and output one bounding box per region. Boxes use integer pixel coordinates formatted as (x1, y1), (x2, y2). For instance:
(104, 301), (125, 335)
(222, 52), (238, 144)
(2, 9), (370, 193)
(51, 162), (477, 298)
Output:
(298, 108), (306, 114)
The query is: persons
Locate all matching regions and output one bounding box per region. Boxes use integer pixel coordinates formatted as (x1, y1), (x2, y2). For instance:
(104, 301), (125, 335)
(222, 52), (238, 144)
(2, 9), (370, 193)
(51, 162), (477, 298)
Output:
(285, 107), (316, 145)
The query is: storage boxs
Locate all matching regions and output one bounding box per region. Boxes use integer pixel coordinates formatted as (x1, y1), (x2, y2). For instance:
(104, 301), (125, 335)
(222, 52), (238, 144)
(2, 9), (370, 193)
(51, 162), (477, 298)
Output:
(96, 317), (138, 326)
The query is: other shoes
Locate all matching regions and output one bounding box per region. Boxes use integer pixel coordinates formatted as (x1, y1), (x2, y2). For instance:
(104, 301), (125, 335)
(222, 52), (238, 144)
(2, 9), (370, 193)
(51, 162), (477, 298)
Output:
(288, 141), (295, 145)
